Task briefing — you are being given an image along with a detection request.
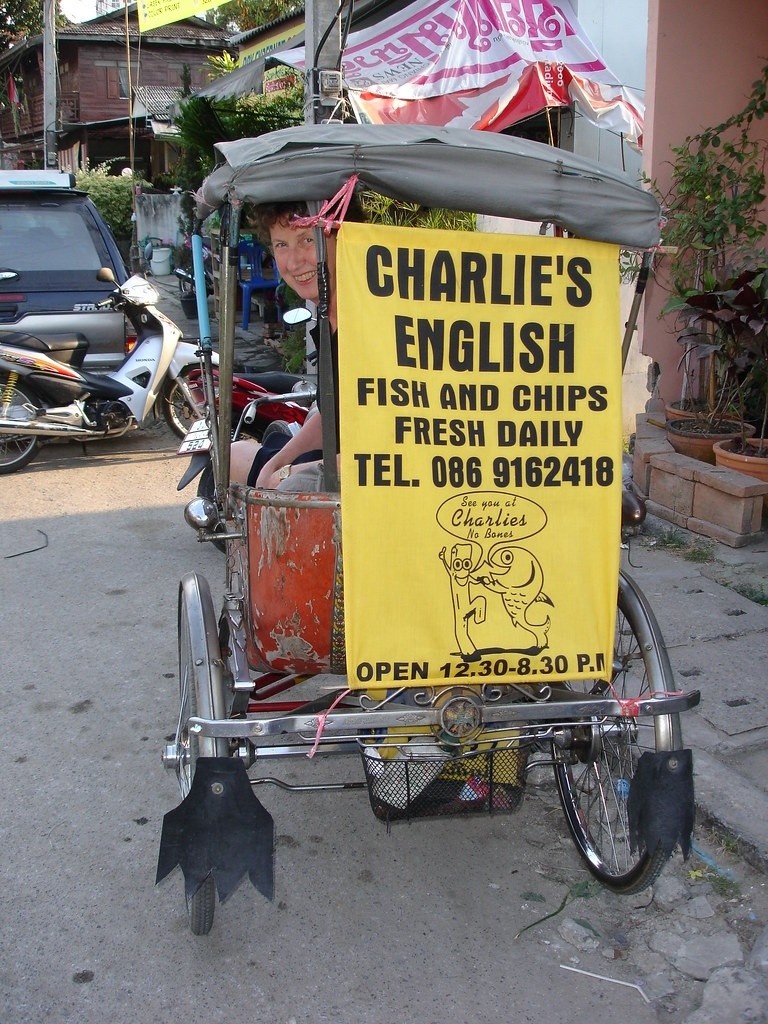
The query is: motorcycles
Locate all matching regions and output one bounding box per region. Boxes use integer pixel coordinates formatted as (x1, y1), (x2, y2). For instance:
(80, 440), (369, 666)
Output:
(0, 243), (234, 477)
(176, 306), (319, 557)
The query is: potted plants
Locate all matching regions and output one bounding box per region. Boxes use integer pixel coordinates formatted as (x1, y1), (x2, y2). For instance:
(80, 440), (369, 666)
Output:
(620, 54), (768, 517)
(176, 176), (209, 319)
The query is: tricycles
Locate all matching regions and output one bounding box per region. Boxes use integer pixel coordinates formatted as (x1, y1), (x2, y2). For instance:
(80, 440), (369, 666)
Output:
(158, 123), (708, 937)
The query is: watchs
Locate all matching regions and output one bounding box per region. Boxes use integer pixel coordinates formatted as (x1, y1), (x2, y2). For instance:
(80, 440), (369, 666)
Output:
(279, 464), (291, 481)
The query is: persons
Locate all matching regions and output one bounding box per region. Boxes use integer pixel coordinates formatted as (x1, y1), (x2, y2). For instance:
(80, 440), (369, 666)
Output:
(231, 197), (368, 493)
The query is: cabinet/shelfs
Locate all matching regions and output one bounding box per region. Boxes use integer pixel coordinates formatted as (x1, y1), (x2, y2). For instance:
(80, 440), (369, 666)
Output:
(210, 228), (274, 321)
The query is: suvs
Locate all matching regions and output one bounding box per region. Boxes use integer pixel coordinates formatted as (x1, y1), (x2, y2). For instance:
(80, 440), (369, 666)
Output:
(0, 168), (138, 377)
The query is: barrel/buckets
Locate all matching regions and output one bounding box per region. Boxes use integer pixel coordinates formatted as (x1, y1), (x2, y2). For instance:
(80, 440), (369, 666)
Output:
(150, 248), (171, 276)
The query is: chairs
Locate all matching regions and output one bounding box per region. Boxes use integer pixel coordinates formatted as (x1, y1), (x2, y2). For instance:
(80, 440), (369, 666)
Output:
(237, 239), (291, 331)
